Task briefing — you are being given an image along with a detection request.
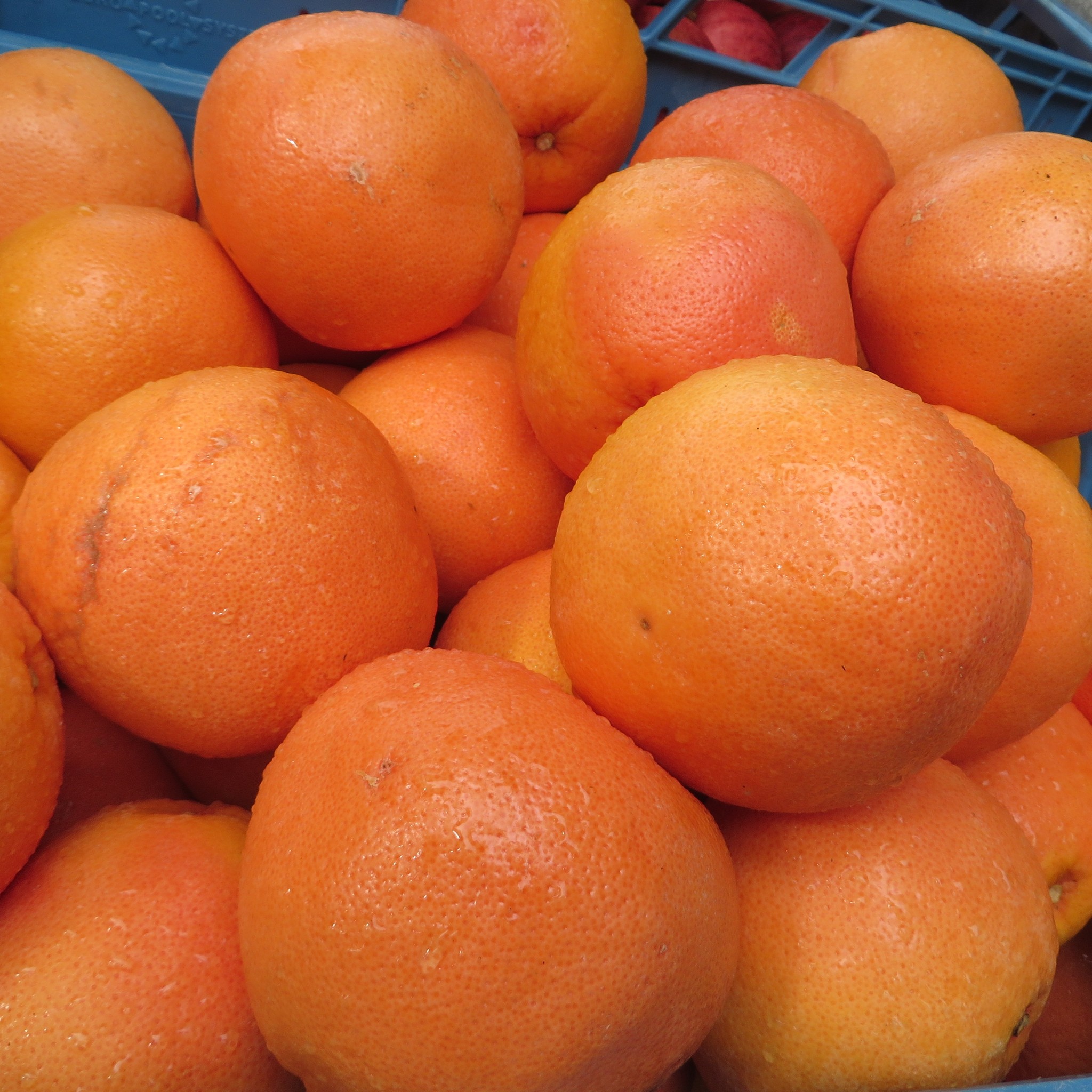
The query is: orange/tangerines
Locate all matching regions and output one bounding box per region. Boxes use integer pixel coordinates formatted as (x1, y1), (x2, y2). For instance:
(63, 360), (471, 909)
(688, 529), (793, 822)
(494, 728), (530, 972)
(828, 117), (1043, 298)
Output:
(0, 0), (1092, 1092)
